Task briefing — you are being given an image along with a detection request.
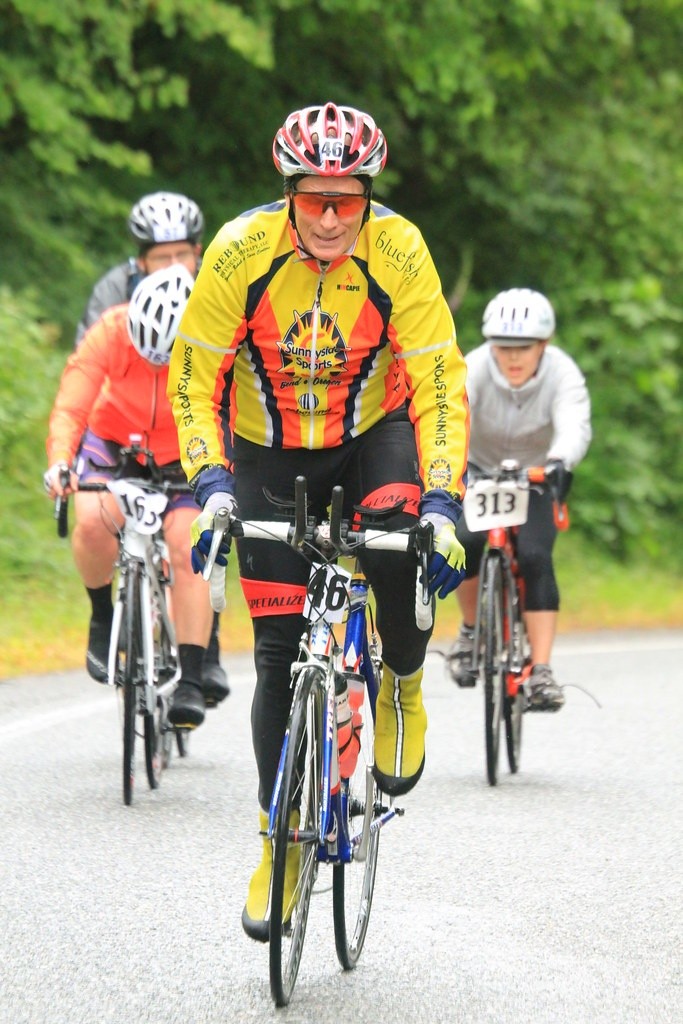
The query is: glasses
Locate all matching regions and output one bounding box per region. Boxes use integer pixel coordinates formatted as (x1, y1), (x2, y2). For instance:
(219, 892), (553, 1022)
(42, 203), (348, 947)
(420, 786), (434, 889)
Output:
(290, 185), (369, 217)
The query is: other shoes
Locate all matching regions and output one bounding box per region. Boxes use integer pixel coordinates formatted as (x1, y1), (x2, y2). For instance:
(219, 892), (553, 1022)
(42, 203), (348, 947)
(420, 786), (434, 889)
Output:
(202, 663), (229, 702)
(84, 616), (119, 682)
(448, 636), (480, 683)
(528, 663), (565, 708)
(169, 677), (206, 727)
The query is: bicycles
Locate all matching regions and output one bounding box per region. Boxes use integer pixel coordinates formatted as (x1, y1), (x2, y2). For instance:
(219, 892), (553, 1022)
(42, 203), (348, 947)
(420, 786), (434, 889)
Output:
(465, 457), (564, 786)
(202, 475), (435, 1011)
(54, 464), (194, 808)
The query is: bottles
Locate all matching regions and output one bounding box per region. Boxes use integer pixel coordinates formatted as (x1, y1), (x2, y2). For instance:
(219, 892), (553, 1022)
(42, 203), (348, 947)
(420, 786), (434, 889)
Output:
(340, 666), (365, 776)
(334, 672), (354, 762)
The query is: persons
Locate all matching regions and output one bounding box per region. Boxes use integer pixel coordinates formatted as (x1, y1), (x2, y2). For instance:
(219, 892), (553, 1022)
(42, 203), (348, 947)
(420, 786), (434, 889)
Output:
(446, 289), (592, 712)
(73, 190), (229, 702)
(166, 100), (471, 940)
(43, 265), (212, 729)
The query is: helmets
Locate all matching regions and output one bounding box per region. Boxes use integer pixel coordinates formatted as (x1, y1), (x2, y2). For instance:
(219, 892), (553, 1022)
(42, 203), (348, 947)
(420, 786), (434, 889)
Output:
(271, 103), (388, 176)
(128, 190), (205, 244)
(480, 286), (556, 347)
(125, 263), (196, 366)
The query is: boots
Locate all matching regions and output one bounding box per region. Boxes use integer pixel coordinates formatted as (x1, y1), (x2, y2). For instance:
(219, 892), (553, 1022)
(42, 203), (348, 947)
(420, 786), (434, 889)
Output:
(241, 807), (302, 943)
(373, 657), (428, 796)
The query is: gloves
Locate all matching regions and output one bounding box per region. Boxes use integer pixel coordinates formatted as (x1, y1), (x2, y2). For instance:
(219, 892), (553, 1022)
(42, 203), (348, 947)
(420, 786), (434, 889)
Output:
(189, 491), (234, 575)
(417, 511), (468, 599)
(546, 467), (574, 501)
